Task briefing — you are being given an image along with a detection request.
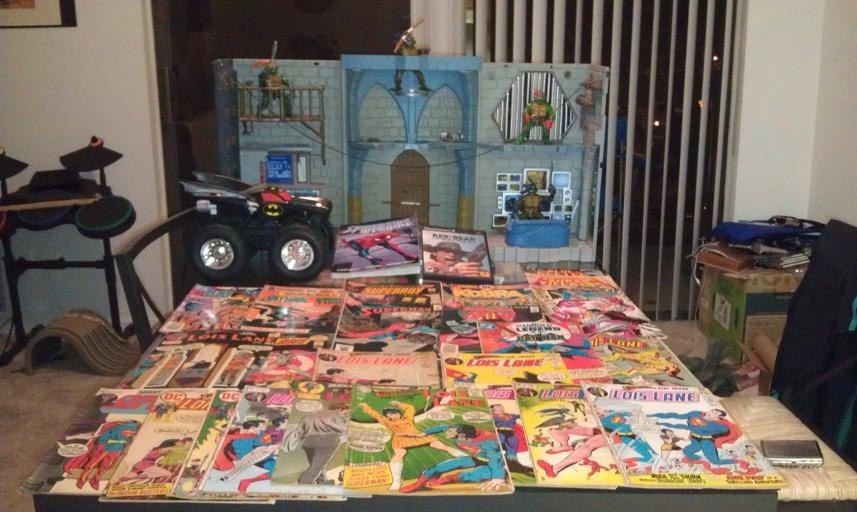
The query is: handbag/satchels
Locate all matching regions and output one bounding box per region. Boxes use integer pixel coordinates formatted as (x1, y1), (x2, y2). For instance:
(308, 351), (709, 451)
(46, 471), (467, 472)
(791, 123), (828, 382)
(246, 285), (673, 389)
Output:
(710, 222), (823, 252)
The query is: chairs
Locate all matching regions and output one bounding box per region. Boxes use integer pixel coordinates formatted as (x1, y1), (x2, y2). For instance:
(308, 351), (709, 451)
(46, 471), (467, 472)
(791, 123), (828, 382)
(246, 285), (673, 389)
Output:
(723, 216), (857, 511)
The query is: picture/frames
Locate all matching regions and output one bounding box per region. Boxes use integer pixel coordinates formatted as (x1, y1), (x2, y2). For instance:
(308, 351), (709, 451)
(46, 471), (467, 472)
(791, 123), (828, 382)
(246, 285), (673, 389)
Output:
(0, 0), (75, 26)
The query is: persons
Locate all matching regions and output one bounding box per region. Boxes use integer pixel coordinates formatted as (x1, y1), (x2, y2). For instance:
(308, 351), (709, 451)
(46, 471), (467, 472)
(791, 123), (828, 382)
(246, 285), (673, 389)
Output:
(389, 18), (435, 94)
(513, 88), (555, 144)
(507, 184), (556, 219)
(255, 39), (296, 120)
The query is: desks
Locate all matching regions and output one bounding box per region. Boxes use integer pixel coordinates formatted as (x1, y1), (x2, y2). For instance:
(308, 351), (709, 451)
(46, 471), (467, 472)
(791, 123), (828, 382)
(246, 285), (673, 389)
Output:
(25, 262), (786, 511)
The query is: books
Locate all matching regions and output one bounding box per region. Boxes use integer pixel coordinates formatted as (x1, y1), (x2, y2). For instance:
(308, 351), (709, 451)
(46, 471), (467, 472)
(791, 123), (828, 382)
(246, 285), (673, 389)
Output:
(419, 225), (494, 285)
(18, 262), (787, 506)
(759, 440), (823, 465)
(694, 239), (810, 273)
(328, 213), (422, 279)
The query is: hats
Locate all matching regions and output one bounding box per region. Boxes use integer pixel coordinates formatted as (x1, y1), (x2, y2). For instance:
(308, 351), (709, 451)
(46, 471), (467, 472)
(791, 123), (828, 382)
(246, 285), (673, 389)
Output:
(423, 242), (473, 258)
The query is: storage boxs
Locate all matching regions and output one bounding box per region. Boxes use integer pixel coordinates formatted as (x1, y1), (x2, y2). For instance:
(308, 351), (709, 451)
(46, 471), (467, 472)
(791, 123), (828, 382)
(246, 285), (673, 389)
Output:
(696, 260), (805, 375)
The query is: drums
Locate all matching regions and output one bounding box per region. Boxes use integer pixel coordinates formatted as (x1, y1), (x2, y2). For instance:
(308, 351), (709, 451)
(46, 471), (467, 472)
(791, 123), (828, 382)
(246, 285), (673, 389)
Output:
(0, 191), (137, 238)
